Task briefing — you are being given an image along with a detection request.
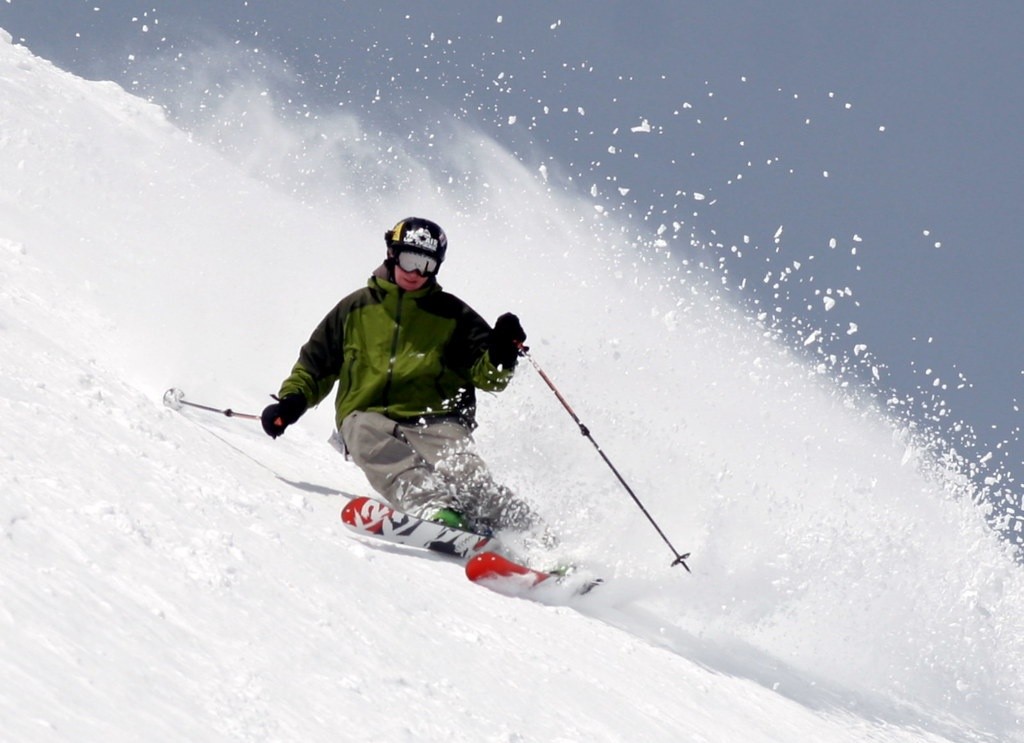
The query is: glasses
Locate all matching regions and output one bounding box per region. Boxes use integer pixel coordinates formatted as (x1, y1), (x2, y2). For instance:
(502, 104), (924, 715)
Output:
(387, 250), (440, 276)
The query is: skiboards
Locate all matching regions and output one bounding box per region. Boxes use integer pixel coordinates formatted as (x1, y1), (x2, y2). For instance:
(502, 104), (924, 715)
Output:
(340, 492), (604, 606)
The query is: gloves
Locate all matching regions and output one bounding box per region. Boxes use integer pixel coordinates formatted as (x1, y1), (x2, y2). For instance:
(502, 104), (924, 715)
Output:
(262, 392), (307, 438)
(494, 313), (528, 369)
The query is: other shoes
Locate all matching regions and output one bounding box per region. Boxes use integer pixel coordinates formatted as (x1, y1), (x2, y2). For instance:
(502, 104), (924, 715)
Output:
(423, 503), (495, 548)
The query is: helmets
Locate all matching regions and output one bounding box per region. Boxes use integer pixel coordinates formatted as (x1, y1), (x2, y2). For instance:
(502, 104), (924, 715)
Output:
(389, 218), (448, 260)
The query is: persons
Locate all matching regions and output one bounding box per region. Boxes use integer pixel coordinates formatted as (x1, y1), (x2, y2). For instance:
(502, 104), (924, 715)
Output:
(262, 217), (577, 576)
